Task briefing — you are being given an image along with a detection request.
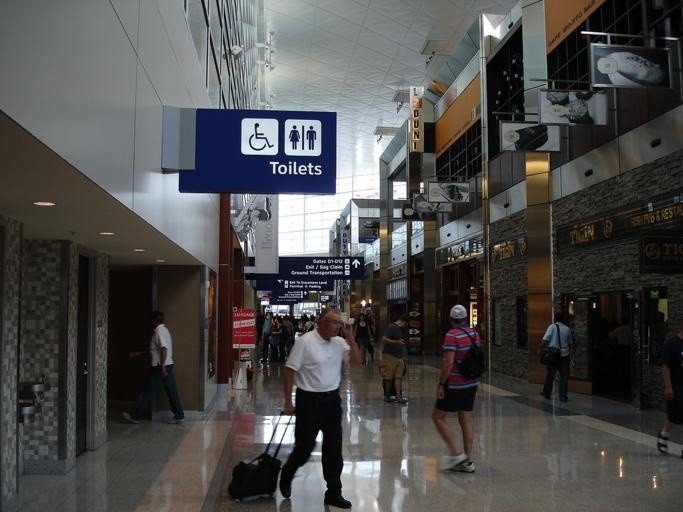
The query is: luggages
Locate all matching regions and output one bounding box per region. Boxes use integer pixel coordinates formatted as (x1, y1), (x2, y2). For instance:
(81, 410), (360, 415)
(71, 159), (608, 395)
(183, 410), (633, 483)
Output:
(228, 410), (292, 502)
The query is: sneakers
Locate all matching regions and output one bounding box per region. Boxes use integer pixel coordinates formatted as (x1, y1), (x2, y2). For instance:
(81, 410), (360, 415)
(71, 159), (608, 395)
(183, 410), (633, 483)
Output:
(279, 464), (291, 499)
(437, 454), (474, 473)
(167, 417), (184, 424)
(324, 490), (352, 509)
(123, 410), (140, 424)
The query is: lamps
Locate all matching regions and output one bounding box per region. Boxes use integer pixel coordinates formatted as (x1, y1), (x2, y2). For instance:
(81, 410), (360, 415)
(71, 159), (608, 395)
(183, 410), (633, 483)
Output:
(396, 96), (406, 113)
(375, 128), (383, 144)
(264, 92), (277, 109)
(263, 29), (276, 73)
(424, 49), (438, 69)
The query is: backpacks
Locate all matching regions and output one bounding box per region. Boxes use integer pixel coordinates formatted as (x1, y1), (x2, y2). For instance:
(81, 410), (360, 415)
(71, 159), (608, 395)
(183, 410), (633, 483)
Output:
(456, 326), (486, 377)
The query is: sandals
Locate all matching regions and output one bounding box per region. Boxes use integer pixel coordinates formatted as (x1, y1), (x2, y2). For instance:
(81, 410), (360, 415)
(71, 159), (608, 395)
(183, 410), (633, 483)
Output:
(656, 431), (668, 453)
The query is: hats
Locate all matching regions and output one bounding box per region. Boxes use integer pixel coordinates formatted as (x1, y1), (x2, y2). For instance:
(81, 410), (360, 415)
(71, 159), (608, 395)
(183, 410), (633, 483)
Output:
(451, 304), (466, 319)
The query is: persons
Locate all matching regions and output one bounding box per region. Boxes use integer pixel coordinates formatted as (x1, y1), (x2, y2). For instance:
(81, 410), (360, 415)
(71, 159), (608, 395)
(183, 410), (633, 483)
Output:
(278, 306), (363, 509)
(122, 311), (184, 424)
(253, 122), (273, 150)
(306, 124), (316, 151)
(430, 304), (483, 473)
(538, 311), (573, 402)
(657, 326), (683, 454)
(288, 125), (299, 151)
(257, 312), (374, 365)
(378, 314), (411, 404)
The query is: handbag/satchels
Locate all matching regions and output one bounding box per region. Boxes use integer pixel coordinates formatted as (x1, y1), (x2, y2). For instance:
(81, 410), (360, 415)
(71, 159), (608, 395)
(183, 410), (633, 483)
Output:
(539, 345), (562, 366)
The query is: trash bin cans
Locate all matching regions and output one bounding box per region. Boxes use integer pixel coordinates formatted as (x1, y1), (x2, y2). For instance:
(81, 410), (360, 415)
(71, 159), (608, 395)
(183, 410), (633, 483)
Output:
(233, 359), (252, 390)
